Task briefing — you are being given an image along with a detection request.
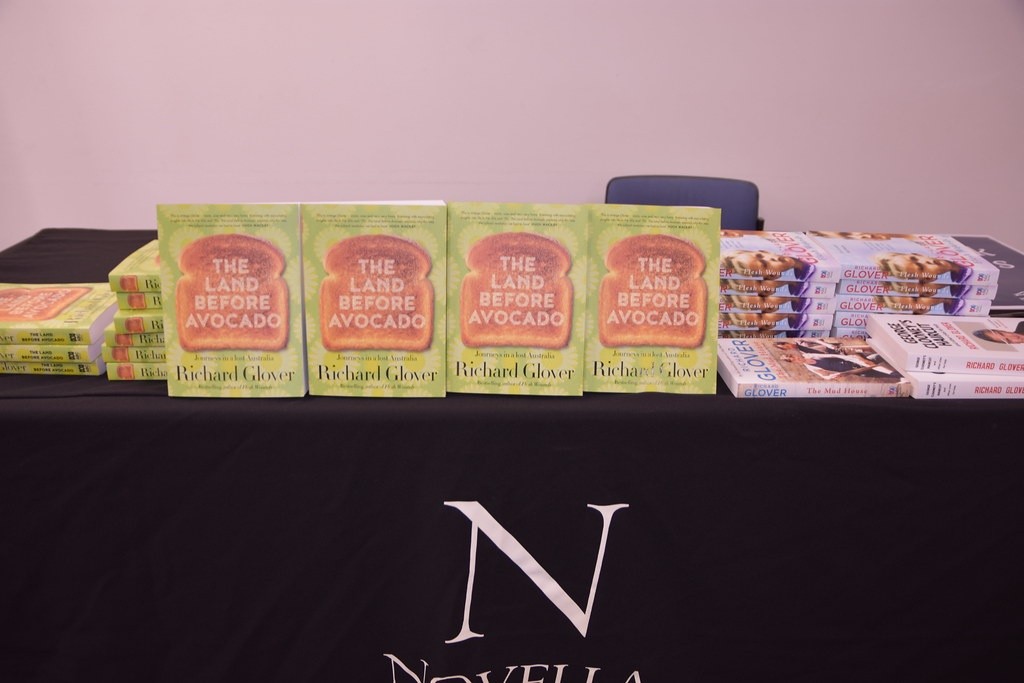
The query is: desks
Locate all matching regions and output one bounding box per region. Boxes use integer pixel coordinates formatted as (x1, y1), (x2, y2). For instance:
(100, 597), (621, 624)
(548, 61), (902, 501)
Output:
(0, 227), (1024, 683)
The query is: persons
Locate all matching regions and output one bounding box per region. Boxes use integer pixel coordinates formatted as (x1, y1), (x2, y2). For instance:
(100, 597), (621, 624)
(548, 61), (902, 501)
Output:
(720, 313), (809, 330)
(721, 230), (769, 239)
(808, 231), (923, 245)
(972, 321), (1024, 345)
(780, 353), (903, 379)
(878, 281), (972, 298)
(774, 339), (852, 355)
(876, 253), (974, 284)
(720, 279), (811, 297)
(718, 330), (807, 338)
(875, 295), (966, 315)
(721, 294), (812, 313)
(720, 250), (815, 282)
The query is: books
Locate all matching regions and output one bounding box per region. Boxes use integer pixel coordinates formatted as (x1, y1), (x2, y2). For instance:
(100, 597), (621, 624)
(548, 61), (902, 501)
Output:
(716, 230), (1024, 398)
(581, 203), (722, 394)
(447, 201), (583, 396)
(156, 201), (308, 397)
(0, 239), (169, 380)
(300, 200), (446, 397)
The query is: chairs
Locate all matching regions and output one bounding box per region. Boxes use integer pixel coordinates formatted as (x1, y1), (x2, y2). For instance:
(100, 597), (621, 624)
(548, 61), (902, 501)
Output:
(605, 175), (765, 231)
